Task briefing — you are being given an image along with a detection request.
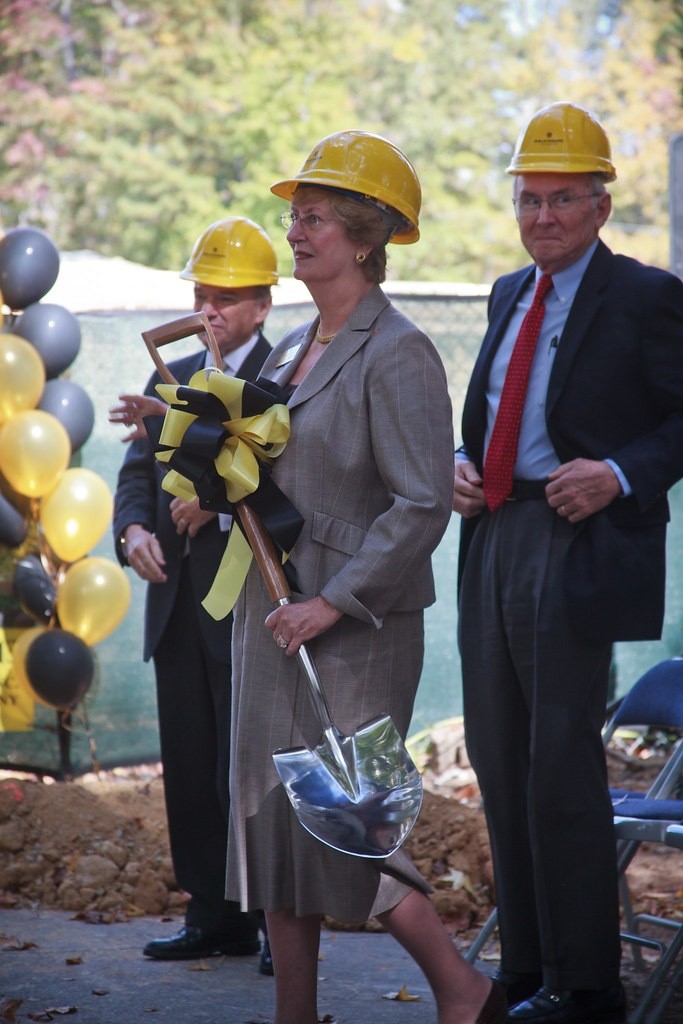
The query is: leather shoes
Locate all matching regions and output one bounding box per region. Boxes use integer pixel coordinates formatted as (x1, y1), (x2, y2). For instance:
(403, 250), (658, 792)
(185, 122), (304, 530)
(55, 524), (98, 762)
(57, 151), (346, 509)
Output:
(142, 923), (262, 959)
(489, 967), (539, 1003)
(260, 936), (273, 975)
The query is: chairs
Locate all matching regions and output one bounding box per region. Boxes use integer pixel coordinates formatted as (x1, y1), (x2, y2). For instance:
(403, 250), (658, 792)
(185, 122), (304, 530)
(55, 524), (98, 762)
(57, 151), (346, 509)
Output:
(462, 653), (683, 1024)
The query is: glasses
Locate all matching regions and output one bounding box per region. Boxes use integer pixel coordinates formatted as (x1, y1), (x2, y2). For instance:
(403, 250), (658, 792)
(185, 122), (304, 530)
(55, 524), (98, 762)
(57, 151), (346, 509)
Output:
(512, 192), (603, 216)
(280, 211), (346, 230)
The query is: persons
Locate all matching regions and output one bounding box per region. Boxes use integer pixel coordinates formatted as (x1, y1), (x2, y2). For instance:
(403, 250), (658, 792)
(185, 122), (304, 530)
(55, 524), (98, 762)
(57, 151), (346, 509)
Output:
(111, 218), (341, 978)
(108, 128), (522, 1024)
(455, 104), (682, 1024)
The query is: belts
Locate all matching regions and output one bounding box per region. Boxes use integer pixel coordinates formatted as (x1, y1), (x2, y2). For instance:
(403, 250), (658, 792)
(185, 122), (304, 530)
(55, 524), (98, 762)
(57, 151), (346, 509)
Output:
(504, 479), (551, 502)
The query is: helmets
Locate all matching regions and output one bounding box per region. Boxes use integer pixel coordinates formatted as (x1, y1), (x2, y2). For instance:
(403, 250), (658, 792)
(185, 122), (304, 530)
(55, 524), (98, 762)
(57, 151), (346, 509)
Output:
(506, 101), (615, 184)
(180, 216), (278, 289)
(270, 130), (422, 244)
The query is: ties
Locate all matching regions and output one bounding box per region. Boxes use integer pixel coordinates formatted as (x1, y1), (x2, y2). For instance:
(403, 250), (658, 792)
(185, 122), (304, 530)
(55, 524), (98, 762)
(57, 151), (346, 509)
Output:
(484, 272), (554, 513)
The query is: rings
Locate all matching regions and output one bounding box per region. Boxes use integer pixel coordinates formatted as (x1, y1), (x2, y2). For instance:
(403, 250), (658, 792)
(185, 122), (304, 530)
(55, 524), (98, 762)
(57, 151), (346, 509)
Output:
(560, 505), (567, 514)
(180, 518), (186, 523)
(276, 634), (289, 647)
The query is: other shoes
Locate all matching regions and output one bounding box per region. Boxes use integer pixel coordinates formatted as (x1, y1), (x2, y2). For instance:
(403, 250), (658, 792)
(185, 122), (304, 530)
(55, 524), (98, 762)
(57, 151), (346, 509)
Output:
(475, 981), (510, 1024)
(506, 984), (627, 1024)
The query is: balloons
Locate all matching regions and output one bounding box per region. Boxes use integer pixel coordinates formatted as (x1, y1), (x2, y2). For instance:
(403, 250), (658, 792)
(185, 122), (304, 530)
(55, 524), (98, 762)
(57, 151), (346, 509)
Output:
(0, 230), (131, 727)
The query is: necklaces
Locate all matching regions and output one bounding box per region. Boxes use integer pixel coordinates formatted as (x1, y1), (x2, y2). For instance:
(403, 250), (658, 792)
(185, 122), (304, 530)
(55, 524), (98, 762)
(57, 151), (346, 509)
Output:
(315, 328), (341, 343)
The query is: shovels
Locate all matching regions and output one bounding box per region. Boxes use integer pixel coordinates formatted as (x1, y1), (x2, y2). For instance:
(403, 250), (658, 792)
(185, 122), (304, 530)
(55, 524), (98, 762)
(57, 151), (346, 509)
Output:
(140, 312), (424, 859)
